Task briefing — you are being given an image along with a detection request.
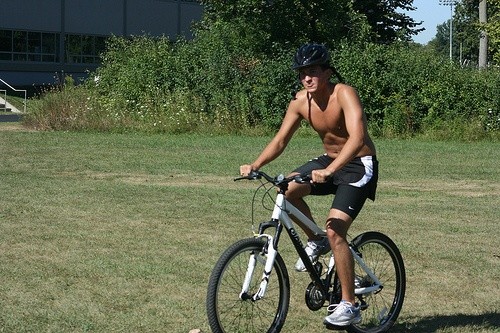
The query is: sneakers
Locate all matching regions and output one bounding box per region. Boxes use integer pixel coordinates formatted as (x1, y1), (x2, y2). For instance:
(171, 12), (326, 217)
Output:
(323, 301), (363, 328)
(295, 229), (331, 272)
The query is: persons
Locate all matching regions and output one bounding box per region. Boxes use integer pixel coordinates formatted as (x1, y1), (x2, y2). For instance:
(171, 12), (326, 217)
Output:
(238, 45), (378, 330)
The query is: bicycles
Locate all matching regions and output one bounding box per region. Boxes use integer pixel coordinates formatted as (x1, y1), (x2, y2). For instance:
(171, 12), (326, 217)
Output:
(206, 168), (406, 333)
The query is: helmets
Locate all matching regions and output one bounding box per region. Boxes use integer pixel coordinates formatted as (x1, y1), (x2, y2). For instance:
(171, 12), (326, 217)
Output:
(291, 43), (332, 70)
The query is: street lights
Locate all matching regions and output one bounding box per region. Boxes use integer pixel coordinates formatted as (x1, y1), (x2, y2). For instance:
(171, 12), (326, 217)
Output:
(439, 0), (461, 63)
